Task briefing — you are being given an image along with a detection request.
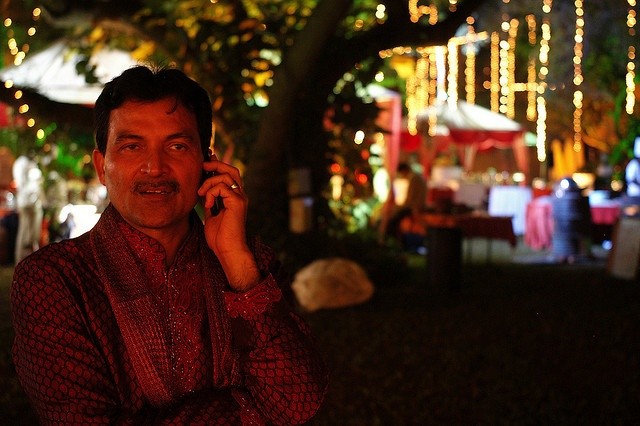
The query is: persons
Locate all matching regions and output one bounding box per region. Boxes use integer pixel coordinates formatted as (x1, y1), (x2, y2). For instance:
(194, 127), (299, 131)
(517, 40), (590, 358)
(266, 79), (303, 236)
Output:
(385, 163), (426, 242)
(9, 66), (331, 426)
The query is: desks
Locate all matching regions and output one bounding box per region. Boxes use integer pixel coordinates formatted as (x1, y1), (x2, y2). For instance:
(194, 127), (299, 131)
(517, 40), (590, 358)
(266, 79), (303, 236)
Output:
(525, 194), (622, 248)
(397, 213), (518, 268)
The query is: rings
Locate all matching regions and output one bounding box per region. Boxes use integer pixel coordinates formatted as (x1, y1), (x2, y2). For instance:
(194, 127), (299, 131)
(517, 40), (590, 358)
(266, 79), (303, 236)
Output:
(230, 182), (240, 188)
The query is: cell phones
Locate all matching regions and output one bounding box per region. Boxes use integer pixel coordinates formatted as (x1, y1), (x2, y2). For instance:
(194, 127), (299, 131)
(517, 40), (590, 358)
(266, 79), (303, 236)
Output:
(205, 153), (221, 216)
